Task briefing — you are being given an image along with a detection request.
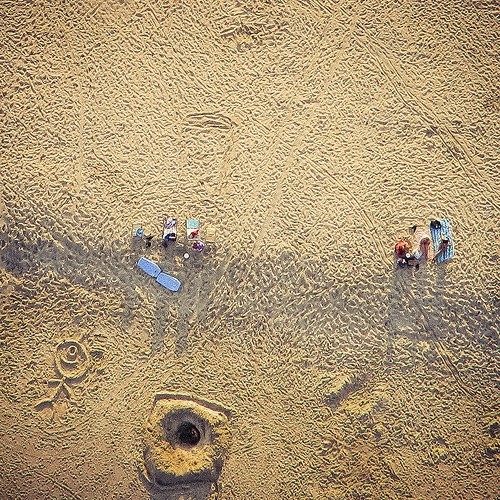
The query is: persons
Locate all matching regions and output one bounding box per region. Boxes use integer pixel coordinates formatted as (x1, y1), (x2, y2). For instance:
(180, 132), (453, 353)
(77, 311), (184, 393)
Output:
(419, 237), (432, 261)
(433, 238), (453, 263)
(395, 241), (411, 259)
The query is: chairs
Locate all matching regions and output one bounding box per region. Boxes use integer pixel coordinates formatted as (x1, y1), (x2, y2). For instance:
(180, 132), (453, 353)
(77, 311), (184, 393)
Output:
(206, 228), (216, 247)
(184, 219), (200, 243)
(130, 223), (142, 241)
(162, 217), (178, 242)
